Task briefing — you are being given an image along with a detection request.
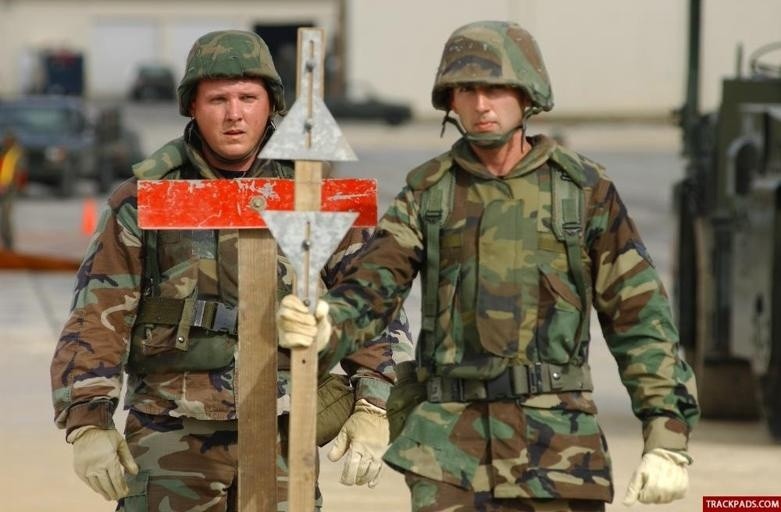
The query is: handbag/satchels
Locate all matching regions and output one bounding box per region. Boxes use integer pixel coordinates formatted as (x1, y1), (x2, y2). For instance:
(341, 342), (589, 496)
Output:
(317, 372), (357, 448)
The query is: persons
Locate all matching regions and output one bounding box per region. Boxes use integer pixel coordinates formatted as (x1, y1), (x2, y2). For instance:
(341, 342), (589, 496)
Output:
(52, 30), (415, 511)
(277, 21), (700, 511)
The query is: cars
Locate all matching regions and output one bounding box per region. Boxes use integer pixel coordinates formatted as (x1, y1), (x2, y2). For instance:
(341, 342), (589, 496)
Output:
(133, 64), (175, 101)
(0, 93), (148, 195)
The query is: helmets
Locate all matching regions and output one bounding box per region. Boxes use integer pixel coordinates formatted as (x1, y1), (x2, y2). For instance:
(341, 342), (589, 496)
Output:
(177, 29), (286, 117)
(432, 20), (554, 112)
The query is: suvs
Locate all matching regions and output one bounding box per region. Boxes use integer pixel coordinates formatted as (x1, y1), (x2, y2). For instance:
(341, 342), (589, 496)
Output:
(660, 0), (781, 441)
(321, 79), (411, 126)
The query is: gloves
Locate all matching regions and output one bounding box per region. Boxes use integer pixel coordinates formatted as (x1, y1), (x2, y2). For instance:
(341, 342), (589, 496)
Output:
(276, 294), (332, 352)
(68, 423), (140, 500)
(623, 447), (691, 505)
(327, 398), (390, 488)
